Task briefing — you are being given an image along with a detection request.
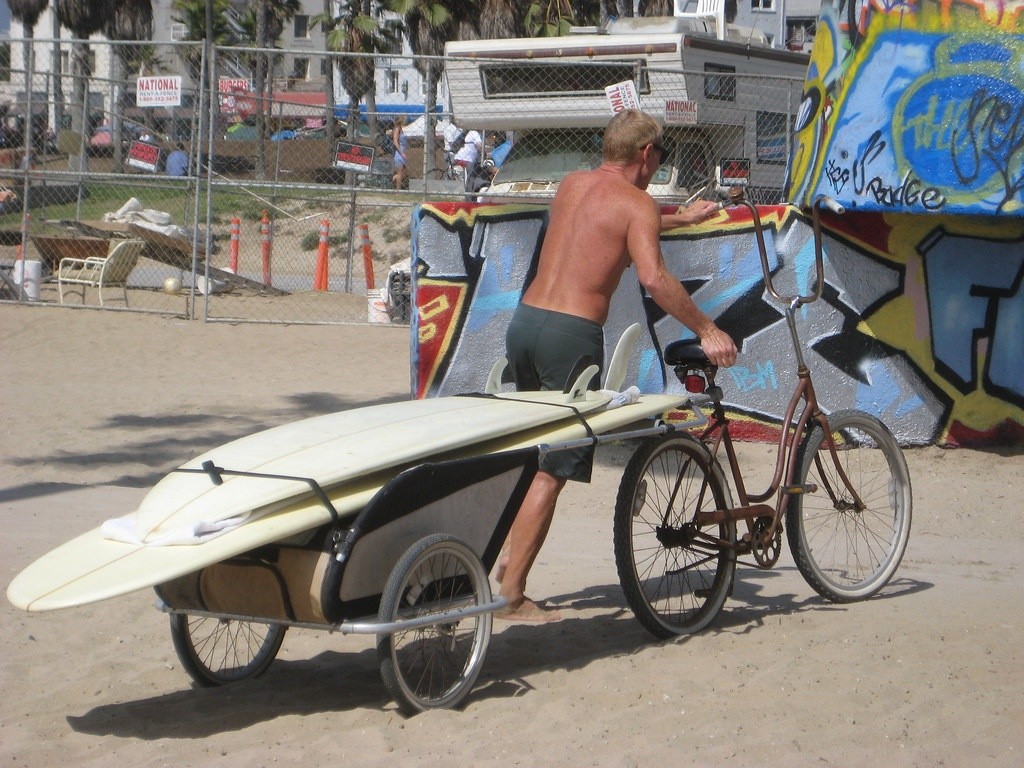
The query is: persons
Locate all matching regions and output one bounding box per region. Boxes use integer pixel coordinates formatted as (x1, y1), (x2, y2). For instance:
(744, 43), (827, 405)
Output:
(139, 131), (151, 142)
(493, 109), (738, 621)
(393, 117), (409, 189)
(444, 119), (497, 202)
(166, 143), (193, 176)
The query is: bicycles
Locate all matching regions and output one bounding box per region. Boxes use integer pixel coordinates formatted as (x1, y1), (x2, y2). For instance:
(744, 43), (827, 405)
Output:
(612, 186), (913, 637)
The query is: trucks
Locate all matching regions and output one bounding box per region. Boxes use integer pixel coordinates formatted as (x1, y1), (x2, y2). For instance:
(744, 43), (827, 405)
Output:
(443, 15), (813, 211)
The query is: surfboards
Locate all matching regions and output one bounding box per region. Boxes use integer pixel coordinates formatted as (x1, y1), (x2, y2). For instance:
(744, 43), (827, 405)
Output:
(1, 320), (709, 616)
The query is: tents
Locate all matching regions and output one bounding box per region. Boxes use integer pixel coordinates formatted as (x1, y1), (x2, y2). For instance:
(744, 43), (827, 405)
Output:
(93, 115), (450, 144)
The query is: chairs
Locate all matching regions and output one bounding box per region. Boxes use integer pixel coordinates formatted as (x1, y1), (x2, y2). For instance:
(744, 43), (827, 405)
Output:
(57, 240), (144, 308)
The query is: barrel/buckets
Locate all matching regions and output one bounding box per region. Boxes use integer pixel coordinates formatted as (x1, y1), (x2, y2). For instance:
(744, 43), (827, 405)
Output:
(14, 260), (40, 301)
(367, 289), (391, 323)
(198, 268), (235, 294)
